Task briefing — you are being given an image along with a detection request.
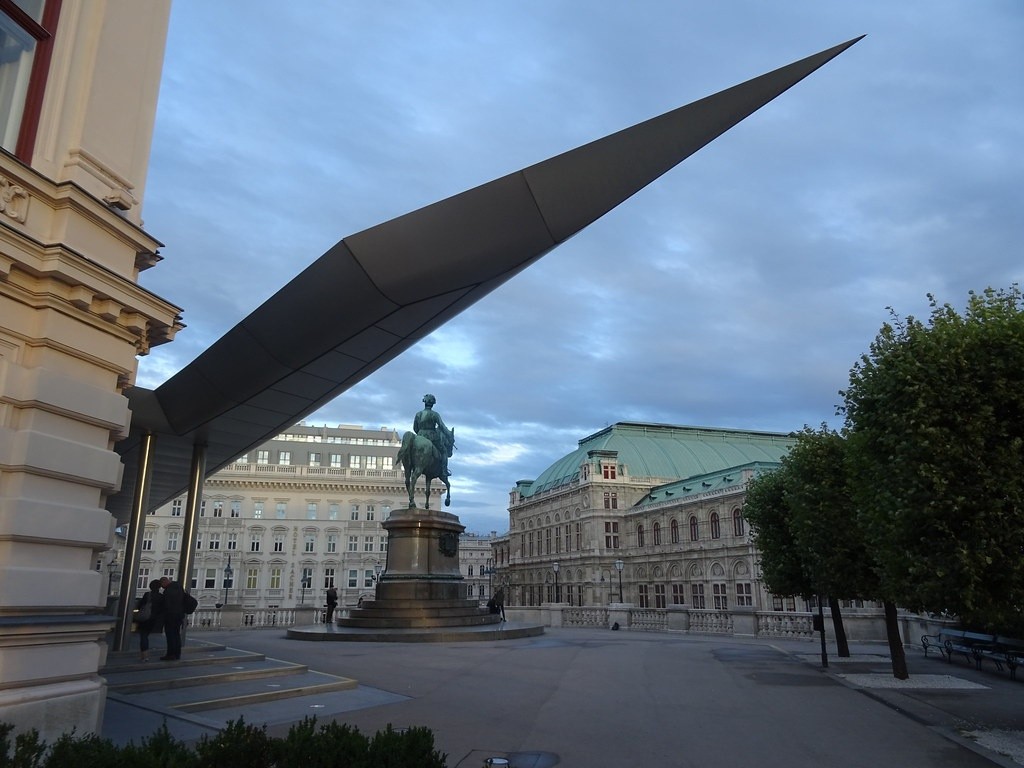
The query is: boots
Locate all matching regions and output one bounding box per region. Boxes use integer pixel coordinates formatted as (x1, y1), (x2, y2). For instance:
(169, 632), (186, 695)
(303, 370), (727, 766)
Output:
(140, 650), (149, 662)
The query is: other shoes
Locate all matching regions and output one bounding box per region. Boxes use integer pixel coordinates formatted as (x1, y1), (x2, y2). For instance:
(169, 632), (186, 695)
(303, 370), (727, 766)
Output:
(160, 654), (176, 660)
(176, 654), (180, 659)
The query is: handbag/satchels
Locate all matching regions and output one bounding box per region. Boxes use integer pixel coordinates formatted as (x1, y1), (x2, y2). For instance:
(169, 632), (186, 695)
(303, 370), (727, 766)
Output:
(134, 591), (152, 622)
(183, 593), (197, 614)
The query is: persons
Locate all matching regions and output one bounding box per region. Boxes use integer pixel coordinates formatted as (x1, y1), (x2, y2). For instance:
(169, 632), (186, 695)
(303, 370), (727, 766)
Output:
(327, 585), (338, 623)
(494, 587), (507, 622)
(133, 577), (183, 661)
(414, 394), (455, 476)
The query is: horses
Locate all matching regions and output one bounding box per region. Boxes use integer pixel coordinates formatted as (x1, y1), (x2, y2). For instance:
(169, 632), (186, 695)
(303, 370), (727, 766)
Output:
(394, 424), (455, 511)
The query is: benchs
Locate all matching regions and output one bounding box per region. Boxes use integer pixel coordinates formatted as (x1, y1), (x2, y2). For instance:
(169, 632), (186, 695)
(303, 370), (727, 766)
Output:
(921, 628), (1024, 679)
